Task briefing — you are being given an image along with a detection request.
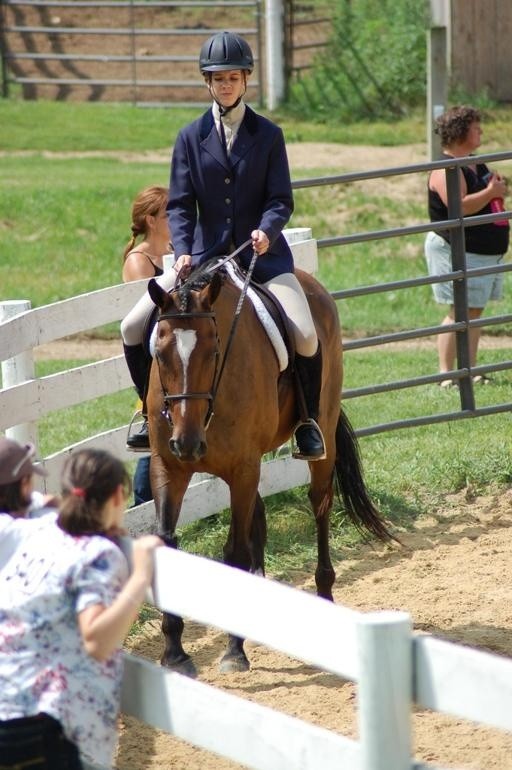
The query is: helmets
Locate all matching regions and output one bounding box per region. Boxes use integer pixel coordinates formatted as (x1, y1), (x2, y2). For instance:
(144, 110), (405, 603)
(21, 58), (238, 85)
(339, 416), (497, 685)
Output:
(200, 33), (254, 72)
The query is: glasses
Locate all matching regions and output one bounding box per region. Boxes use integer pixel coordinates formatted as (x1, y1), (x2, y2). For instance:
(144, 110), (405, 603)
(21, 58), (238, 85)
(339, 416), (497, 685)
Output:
(11, 443), (36, 477)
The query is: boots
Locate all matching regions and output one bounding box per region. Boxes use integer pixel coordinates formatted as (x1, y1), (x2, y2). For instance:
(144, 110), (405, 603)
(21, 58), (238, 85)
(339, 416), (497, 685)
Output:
(293, 338), (324, 456)
(123, 344), (153, 448)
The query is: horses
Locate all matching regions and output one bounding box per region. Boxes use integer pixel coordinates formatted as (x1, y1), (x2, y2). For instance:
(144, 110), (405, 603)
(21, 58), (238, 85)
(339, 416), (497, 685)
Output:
(146, 268), (409, 675)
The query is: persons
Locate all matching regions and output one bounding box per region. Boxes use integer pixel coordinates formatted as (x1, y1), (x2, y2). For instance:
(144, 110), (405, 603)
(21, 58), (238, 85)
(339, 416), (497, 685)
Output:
(1, 435), (62, 583)
(117, 186), (174, 506)
(421, 102), (512, 393)
(110, 26), (327, 464)
(1, 448), (169, 770)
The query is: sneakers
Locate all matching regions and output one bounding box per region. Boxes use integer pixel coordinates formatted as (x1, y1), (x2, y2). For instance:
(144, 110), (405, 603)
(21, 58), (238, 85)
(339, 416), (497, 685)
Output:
(439, 379), (458, 389)
(472, 375), (493, 386)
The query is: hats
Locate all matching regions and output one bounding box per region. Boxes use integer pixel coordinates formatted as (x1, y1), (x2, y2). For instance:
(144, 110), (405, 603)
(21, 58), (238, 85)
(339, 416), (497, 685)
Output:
(1, 440), (50, 484)
